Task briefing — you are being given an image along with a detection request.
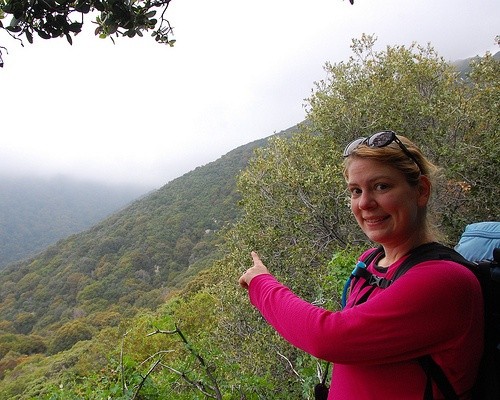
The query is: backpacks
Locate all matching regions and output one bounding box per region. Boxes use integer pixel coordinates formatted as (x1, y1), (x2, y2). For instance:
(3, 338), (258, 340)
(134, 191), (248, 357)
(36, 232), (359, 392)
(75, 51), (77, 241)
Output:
(353, 222), (500, 399)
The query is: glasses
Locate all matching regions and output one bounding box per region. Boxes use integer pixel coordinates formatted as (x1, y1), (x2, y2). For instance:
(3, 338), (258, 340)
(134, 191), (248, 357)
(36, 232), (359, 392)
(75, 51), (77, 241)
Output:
(342, 130), (425, 174)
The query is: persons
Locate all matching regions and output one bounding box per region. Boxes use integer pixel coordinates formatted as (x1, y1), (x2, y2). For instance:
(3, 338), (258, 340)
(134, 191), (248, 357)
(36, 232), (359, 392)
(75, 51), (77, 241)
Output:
(239, 131), (500, 400)
